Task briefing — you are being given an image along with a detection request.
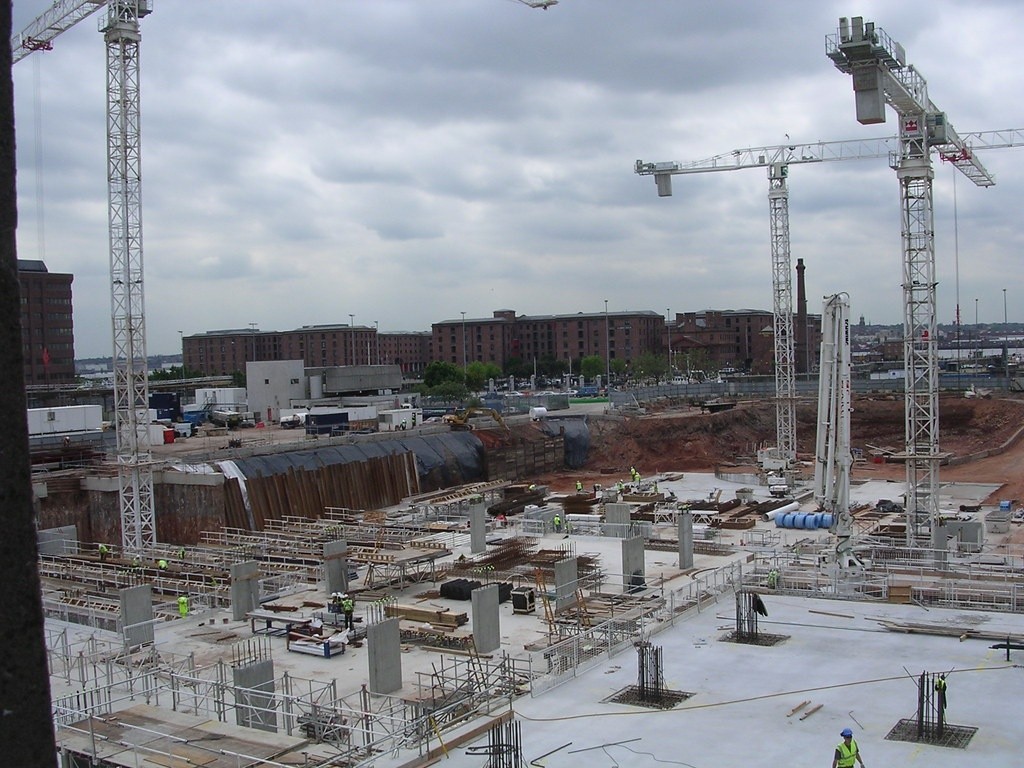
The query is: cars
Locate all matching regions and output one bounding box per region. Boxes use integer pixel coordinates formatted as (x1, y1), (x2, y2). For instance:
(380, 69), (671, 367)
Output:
(561, 390), (577, 397)
(506, 391), (526, 399)
(534, 391), (554, 396)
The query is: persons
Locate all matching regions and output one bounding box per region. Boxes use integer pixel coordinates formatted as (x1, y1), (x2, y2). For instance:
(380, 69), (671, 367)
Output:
(635, 472), (641, 481)
(630, 466), (636, 482)
(618, 479), (625, 495)
(593, 483), (602, 493)
(831, 729), (866, 768)
(528, 483), (537, 491)
(341, 596), (355, 630)
(178, 594), (188, 618)
(99, 544), (108, 560)
(177, 546), (185, 560)
(766, 567), (780, 589)
(576, 480), (583, 492)
(554, 514), (562, 533)
(156, 559), (169, 571)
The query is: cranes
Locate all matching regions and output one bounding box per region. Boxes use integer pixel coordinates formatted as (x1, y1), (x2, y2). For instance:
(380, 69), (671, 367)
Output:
(11, 0), (158, 556)
(631, 128), (1023, 475)
(825, 14), (997, 548)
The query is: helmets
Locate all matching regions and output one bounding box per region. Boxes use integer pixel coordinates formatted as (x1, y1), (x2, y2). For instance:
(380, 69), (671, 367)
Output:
(772, 572), (776, 575)
(620, 479), (624, 483)
(331, 591), (349, 601)
(840, 728), (854, 736)
(555, 514), (560, 516)
(636, 472), (639, 475)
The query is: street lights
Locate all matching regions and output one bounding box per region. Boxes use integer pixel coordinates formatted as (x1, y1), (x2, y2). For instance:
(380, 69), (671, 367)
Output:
(1003, 289), (1010, 380)
(348, 313), (356, 365)
(666, 308), (671, 374)
(604, 299), (611, 395)
(975, 298), (978, 382)
(374, 321), (383, 364)
(461, 310), (467, 383)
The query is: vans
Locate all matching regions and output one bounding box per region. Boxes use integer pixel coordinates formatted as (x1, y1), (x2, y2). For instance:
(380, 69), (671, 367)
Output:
(576, 387), (599, 399)
